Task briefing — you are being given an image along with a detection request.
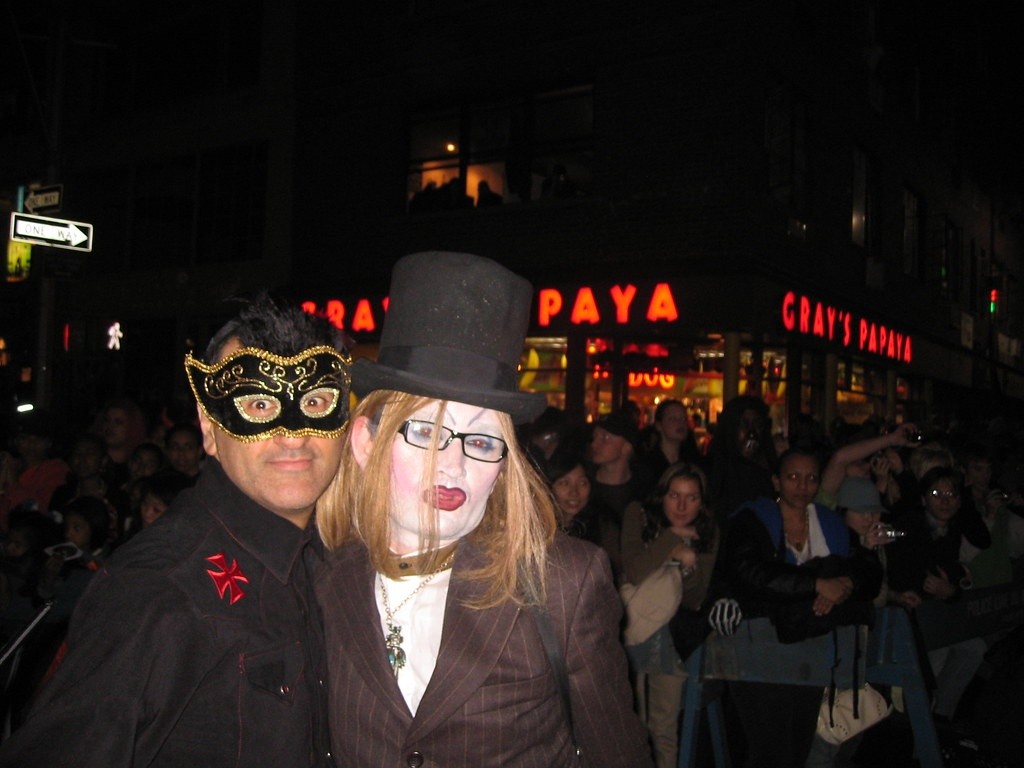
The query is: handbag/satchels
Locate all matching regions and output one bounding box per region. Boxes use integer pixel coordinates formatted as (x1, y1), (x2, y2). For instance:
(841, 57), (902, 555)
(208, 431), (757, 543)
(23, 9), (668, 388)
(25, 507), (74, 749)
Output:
(818, 682), (893, 745)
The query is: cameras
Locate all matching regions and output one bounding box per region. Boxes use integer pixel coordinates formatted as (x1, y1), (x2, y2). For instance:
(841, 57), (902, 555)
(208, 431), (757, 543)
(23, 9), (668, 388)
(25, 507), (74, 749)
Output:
(876, 525), (896, 541)
(905, 428), (924, 442)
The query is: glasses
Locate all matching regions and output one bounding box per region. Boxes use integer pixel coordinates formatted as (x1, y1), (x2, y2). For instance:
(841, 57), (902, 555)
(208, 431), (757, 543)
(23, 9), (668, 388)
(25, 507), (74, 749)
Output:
(373, 419), (509, 463)
(923, 488), (959, 500)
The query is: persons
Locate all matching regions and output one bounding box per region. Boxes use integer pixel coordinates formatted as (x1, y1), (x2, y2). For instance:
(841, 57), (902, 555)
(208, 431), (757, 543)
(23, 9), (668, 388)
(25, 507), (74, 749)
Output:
(410, 179), (502, 215)
(0, 290), (354, 768)
(0, 362), (1024, 768)
(289, 250), (662, 768)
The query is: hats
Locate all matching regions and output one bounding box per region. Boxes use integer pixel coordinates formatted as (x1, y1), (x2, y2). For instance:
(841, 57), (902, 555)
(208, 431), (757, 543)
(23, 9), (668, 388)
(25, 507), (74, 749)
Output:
(834, 477), (891, 515)
(592, 410), (639, 447)
(346, 251), (548, 424)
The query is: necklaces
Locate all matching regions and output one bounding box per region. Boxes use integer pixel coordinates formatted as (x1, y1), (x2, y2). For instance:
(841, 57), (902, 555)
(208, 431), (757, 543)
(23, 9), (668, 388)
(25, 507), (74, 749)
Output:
(363, 542), (476, 681)
(783, 510), (808, 551)
